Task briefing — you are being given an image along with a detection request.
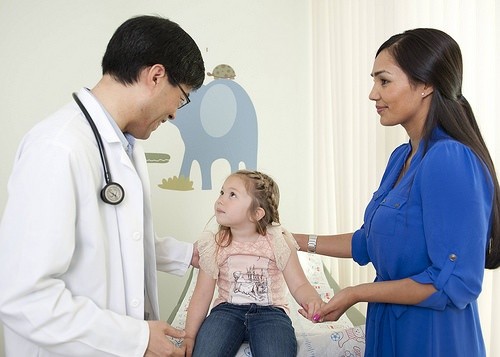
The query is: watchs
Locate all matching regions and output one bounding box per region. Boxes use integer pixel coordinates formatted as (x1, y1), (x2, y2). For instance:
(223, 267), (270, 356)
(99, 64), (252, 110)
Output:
(307, 233), (318, 255)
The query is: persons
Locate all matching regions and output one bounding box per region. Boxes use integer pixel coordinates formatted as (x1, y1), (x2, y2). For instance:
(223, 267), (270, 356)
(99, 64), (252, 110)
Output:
(1, 0), (205, 357)
(178, 168), (327, 357)
(292, 27), (500, 357)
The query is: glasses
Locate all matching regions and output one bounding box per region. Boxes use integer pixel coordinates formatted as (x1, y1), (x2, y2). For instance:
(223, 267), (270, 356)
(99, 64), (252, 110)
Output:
(166, 68), (191, 110)
